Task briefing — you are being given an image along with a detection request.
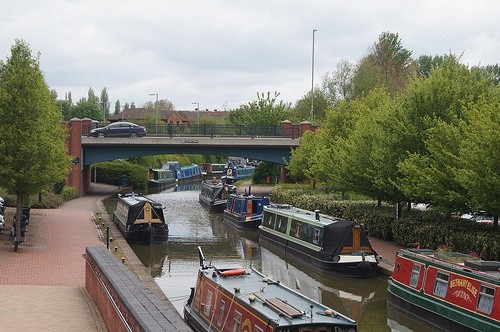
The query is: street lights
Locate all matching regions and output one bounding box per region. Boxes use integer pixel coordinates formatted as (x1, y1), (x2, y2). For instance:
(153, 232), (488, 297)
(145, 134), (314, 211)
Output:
(148, 93), (159, 124)
(192, 102), (200, 124)
(309, 28), (321, 126)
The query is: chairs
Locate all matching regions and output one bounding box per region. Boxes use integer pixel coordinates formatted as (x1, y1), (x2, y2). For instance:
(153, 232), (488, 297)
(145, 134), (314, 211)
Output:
(9, 219), (27, 252)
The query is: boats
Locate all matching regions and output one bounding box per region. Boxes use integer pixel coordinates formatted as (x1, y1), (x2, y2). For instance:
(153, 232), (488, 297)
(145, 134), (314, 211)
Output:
(160, 162), (180, 174)
(201, 164), (229, 176)
(224, 186), (272, 230)
(385, 246), (500, 332)
(202, 163), (212, 171)
(149, 169), (176, 184)
(221, 157), (263, 183)
(177, 165), (201, 179)
(258, 196), (382, 279)
(113, 190), (171, 242)
(182, 239), (358, 332)
(199, 180), (237, 210)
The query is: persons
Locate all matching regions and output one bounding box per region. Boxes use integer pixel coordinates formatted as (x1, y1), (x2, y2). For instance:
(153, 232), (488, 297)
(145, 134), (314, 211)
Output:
(168, 121), (173, 138)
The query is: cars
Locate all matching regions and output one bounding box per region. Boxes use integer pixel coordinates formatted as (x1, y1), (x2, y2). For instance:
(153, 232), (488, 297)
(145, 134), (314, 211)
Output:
(460, 210), (500, 224)
(406, 201), (432, 211)
(0, 214), (5, 228)
(0, 197), (5, 213)
(90, 121), (147, 138)
